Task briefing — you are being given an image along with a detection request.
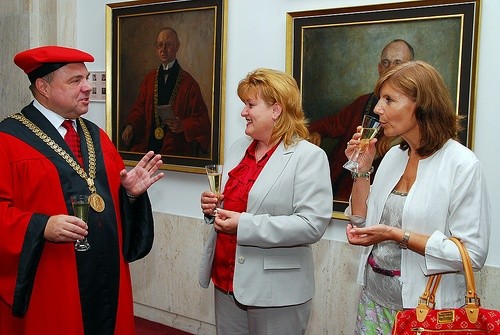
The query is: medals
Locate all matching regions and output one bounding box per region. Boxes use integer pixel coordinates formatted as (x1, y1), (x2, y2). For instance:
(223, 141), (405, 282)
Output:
(88, 194), (105, 212)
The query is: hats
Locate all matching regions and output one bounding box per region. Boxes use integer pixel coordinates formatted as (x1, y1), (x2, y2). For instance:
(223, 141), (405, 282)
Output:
(13, 46), (95, 80)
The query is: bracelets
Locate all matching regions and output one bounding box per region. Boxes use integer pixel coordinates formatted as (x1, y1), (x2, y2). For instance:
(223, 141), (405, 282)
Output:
(399, 231), (410, 249)
(351, 166), (374, 182)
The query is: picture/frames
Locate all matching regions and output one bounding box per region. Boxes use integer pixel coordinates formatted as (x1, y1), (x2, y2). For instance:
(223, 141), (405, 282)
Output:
(105, 0), (228, 176)
(285, 0), (483, 221)
(89, 71), (105, 102)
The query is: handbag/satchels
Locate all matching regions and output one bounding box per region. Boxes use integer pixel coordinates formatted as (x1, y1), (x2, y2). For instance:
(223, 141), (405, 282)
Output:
(391, 237), (500, 335)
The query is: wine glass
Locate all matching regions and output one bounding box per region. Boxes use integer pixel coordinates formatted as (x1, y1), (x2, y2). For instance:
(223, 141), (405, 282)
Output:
(343, 115), (383, 174)
(70, 195), (90, 252)
(205, 165), (224, 216)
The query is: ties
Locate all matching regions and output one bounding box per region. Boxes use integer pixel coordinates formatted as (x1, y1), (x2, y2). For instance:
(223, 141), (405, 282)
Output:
(61, 120), (86, 172)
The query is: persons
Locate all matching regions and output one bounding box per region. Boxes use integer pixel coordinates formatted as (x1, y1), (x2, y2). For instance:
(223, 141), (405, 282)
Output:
(0, 45), (165, 335)
(198, 67), (334, 335)
(344, 61), (491, 335)
